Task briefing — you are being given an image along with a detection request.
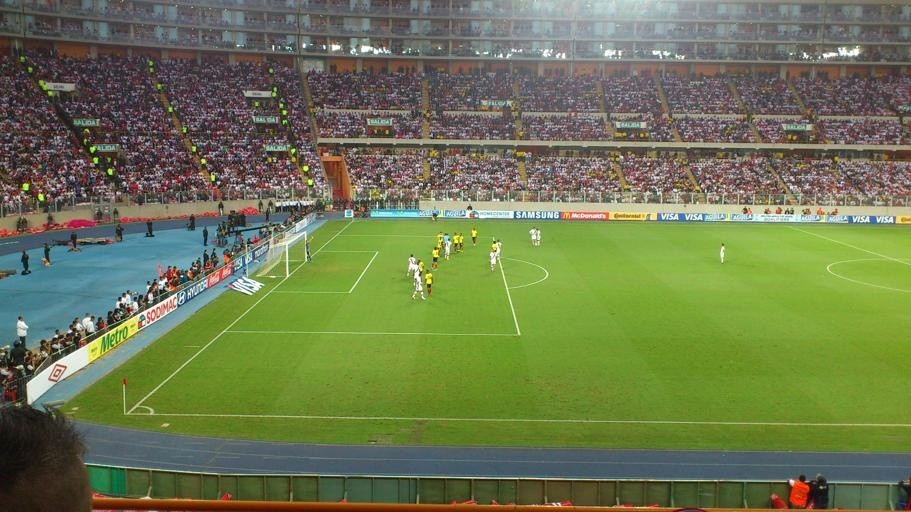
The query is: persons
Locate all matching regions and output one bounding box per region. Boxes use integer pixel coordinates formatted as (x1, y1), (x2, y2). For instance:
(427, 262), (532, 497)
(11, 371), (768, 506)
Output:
(1, 44), (911, 221)
(811, 473), (829, 510)
(1, 199), (325, 406)
(529, 226), (541, 247)
(720, 243), (726, 263)
(407, 254), (433, 301)
(1, 402), (93, 510)
(789, 473), (809, 509)
(471, 228), (477, 246)
(431, 231), (465, 269)
(489, 239), (502, 271)
